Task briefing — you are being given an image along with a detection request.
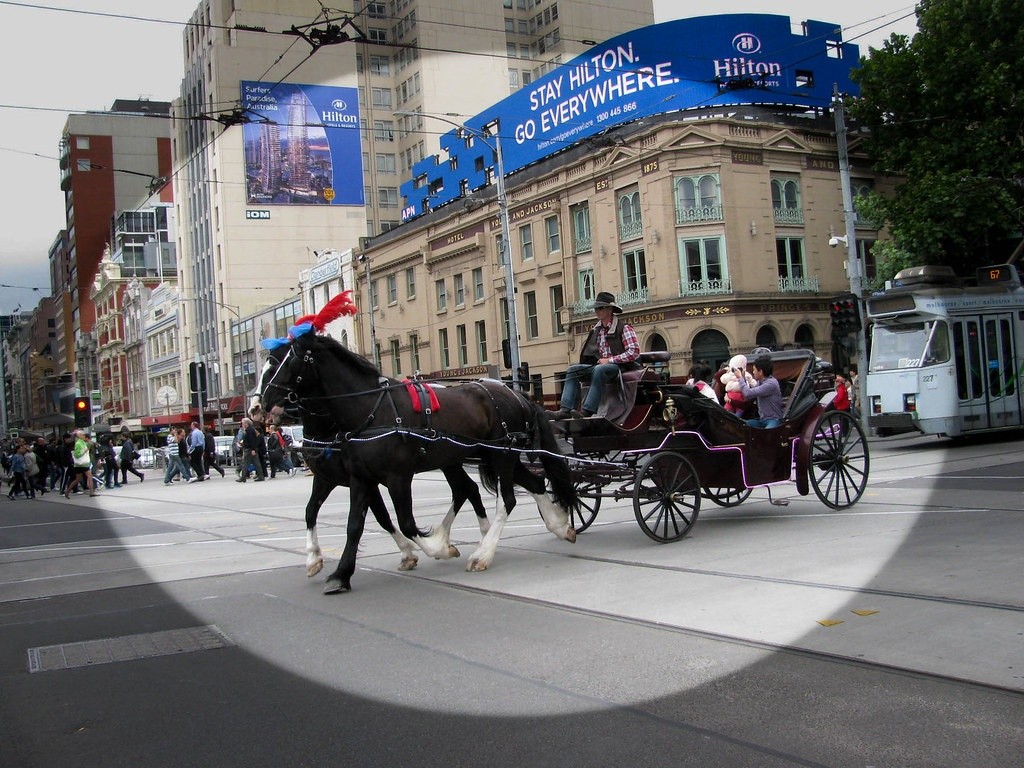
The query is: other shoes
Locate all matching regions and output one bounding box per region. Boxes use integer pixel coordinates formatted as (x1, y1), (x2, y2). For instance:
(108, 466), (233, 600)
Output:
(570, 410), (583, 418)
(236, 478), (246, 482)
(267, 476), (275, 480)
(141, 473), (144, 482)
(222, 469), (225, 477)
(164, 474), (210, 486)
(292, 468), (297, 475)
(545, 409), (571, 419)
(289, 469), (293, 477)
(253, 476), (258, 479)
(7, 482), (128, 500)
(254, 479), (264, 481)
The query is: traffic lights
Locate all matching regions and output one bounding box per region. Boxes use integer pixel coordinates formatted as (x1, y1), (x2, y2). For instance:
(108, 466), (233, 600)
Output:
(74, 397), (91, 428)
(517, 362), (530, 391)
(829, 293), (862, 334)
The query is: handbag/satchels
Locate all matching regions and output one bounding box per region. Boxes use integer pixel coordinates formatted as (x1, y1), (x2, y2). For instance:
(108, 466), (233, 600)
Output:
(133, 451), (141, 460)
(35, 456), (45, 469)
(97, 458), (106, 466)
(72, 440), (91, 467)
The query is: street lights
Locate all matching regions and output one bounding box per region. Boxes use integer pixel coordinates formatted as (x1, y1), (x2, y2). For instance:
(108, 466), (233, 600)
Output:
(393, 110), (520, 391)
(177, 298), (246, 418)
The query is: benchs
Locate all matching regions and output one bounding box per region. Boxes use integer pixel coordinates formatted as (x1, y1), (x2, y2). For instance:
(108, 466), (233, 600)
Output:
(582, 351), (671, 387)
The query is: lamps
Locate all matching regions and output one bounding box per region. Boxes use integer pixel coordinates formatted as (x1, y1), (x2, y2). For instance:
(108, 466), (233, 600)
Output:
(399, 304), (404, 314)
(446, 290), (451, 300)
(380, 310), (385, 319)
(599, 243), (605, 258)
(464, 284), (469, 296)
(651, 229), (659, 246)
(750, 220), (757, 236)
(828, 224), (835, 238)
(533, 263), (542, 277)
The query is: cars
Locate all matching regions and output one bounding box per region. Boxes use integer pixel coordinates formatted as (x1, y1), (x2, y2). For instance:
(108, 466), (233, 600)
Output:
(213, 436), (237, 466)
(266, 426), (304, 467)
(133, 446), (169, 469)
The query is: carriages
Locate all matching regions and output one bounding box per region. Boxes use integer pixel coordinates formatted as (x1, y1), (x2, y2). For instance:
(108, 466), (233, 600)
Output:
(248, 290), (870, 594)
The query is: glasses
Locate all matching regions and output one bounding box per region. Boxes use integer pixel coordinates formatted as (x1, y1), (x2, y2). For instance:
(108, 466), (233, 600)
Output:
(595, 306), (609, 310)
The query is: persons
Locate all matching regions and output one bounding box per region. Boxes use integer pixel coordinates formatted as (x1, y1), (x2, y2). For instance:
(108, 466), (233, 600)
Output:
(846, 378), (855, 417)
(119, 432), (145, 484)
(833, 375), (850, 438)
(236, 418), (266, 482)
(731, 355), (781, 428)
(546, 292), (640, 420)
(64, 430), (100, 498)
(849, 366), (861, 411)
(686, 363), (720, 404)
(190, 421), (211, 483)
(720, 355), (758, 417)
(711, 358), (731, 405)
(0, 425), (314, 500)
(164, 425), (197, 486)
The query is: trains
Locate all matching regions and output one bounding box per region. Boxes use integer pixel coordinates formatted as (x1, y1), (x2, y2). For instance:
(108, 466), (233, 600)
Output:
(866, 263), (1024, 438)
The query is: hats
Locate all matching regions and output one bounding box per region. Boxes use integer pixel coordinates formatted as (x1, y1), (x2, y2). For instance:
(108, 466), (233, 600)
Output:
(729, 354), (747, 373)
(834, 376), (845, 384)
(586, 291), (623, 313)
(848, 368), (858, 374)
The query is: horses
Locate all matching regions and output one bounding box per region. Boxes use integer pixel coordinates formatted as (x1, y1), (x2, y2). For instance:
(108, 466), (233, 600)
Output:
(246, 322), (580, 594)
